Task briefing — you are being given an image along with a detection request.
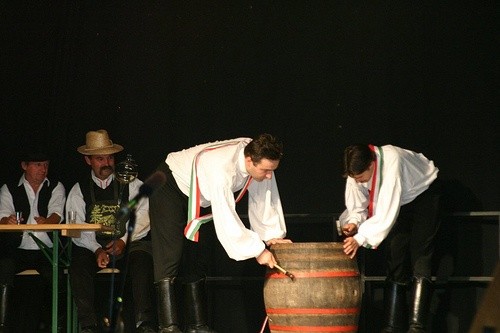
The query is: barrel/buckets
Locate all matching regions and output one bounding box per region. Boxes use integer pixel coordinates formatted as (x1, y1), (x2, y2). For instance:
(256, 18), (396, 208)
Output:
(263, 242), (362, 333)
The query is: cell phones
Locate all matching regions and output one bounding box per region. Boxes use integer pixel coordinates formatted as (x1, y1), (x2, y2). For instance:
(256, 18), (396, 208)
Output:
(17, 211), (21, 225)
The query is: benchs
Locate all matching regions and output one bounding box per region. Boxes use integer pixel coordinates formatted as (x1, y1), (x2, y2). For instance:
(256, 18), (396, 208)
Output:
(0, 264), (120, 333)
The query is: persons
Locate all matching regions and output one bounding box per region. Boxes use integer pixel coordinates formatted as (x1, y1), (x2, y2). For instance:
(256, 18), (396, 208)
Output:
(333, 142), (443, 333)
(66, 129), (156, 333)
(0, 150), (65, 333)
(149, 132), (292, 332)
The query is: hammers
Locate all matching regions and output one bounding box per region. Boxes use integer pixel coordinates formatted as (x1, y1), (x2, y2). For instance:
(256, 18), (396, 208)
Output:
(273, 263), (295, 280)
(336, 220), (348, 236)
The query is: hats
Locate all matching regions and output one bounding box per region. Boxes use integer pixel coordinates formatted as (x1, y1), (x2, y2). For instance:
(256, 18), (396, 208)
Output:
(18, 142), (50, 162)
(78, 130), (124, 155)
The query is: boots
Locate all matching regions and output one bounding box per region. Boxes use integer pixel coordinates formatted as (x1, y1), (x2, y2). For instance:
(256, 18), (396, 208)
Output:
(378, 279), (408, 333)
(403, 275), (434, 333)
(180, 279), (218, 333)
(153, 277), (185, 333)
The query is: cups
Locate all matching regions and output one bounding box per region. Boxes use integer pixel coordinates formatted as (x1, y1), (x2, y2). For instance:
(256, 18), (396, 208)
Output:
(15, 211), (22, 224)
(68, 211), (77, 224)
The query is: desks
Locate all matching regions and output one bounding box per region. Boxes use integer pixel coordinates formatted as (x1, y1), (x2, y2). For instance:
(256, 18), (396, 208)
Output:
(0, 223), (114, 333)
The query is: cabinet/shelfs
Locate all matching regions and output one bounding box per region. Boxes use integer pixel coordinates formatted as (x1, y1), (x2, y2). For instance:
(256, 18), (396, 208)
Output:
(199, 209), (500, 284)
